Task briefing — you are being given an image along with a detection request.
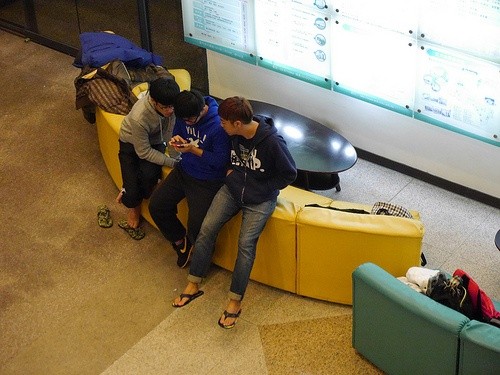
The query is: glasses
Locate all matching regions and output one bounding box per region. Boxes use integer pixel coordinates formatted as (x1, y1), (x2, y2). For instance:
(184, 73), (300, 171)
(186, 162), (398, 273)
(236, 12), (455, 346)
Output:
(157, 103), (173, 110)
(182, 113), (201, 125)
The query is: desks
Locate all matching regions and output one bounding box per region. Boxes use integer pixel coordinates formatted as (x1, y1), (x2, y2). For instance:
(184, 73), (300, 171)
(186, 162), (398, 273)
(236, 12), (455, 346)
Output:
(247, 99), (358, 192)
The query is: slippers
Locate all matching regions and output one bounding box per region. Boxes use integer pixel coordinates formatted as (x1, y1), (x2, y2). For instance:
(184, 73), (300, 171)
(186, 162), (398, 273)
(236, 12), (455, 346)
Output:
(118, 218), (145, 240)
(172, 290), (204, 307)
(96, 203), (113, 228)
(218, 309), (242, 329)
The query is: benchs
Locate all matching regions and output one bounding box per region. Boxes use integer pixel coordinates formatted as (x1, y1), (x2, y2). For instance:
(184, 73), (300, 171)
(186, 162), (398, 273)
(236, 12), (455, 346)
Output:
(94, 68), (424, 306)
(351, 261), (500, 375)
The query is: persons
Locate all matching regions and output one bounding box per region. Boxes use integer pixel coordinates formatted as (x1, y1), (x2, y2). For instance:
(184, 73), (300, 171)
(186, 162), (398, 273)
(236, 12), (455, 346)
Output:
(148, 88), (228, 268)
(172, 96), (297, 329)
(116, 76), (180, 227)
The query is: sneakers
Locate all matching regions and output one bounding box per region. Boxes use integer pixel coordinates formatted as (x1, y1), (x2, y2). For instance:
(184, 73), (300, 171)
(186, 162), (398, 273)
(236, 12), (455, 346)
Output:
(172, 234), (194, 269)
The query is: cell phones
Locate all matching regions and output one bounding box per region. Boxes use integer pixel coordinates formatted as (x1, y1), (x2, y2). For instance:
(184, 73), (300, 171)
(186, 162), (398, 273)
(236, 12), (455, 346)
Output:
(172, 142), (191, 147)
